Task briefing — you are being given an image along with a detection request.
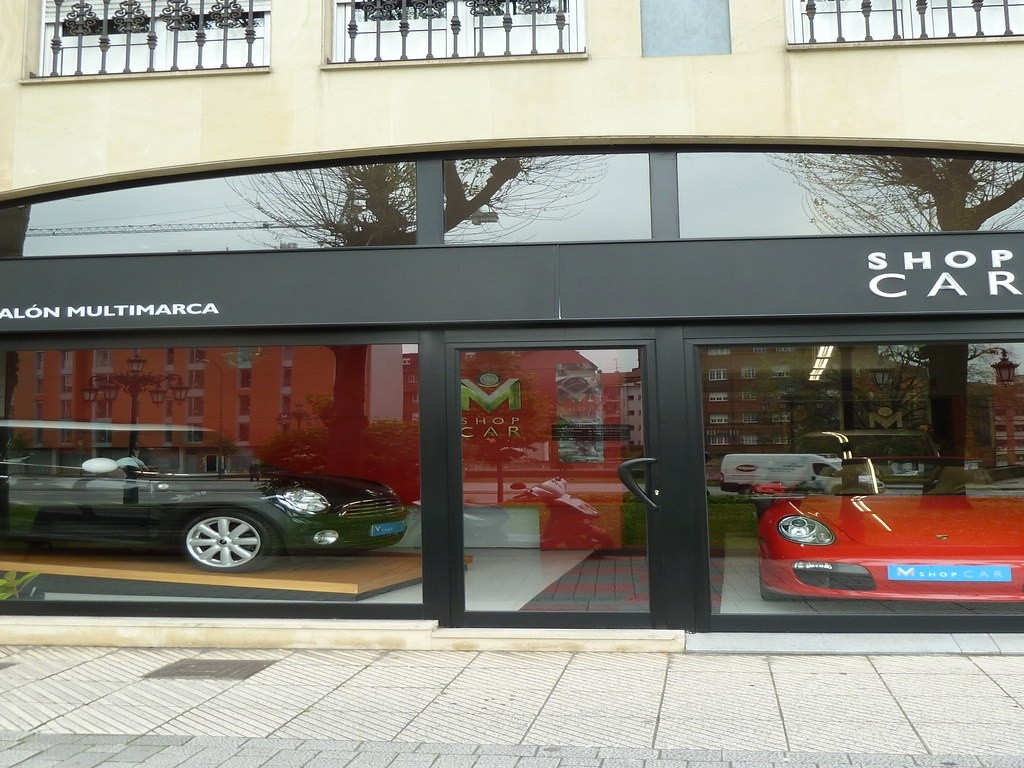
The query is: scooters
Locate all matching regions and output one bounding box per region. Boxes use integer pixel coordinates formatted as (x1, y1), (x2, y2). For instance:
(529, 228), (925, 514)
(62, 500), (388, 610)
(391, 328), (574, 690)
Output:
(412, 467), (616, 550)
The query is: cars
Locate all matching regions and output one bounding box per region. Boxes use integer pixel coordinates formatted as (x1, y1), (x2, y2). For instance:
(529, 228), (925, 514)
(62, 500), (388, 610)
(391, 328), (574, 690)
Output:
(783, 428), (947, 494)
(756, 455), (1024, 603)
(0, 419), (411, 574)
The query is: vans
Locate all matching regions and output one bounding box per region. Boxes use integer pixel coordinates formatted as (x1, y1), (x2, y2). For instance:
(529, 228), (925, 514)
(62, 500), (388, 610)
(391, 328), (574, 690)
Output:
(719, 453), (886, 495)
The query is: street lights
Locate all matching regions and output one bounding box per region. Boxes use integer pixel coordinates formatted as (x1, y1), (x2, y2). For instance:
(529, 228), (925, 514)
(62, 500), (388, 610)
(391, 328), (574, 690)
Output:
(202, 359), (223, 473)
(82, 355), (191, 505)
